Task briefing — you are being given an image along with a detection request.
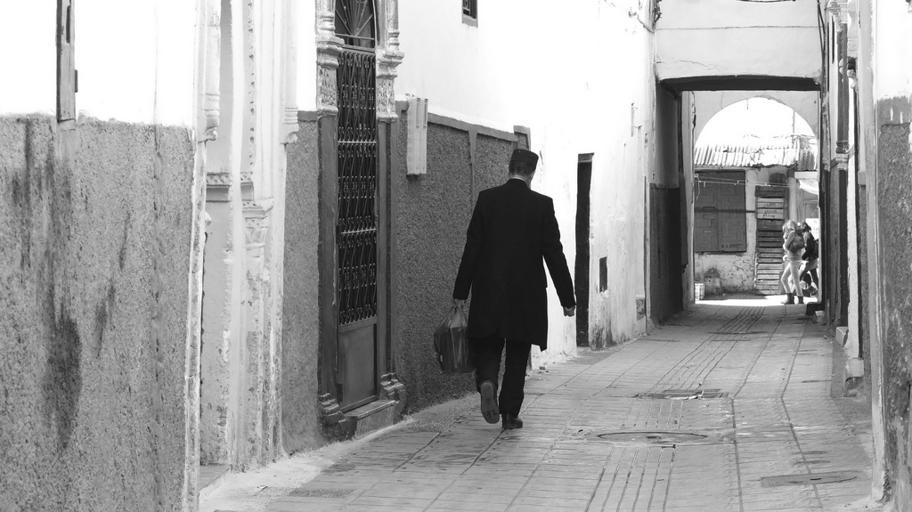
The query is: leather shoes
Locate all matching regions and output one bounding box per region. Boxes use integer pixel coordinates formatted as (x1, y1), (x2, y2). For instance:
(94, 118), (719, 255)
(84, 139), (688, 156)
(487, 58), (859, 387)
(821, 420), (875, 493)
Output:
(502, 415), (522, 428)
(480, 381), (499, 423)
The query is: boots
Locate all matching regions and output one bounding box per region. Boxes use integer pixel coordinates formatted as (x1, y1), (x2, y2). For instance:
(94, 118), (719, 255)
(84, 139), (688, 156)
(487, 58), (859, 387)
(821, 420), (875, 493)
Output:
(798, 296), (803, 304)
(784, 293), (794, 304)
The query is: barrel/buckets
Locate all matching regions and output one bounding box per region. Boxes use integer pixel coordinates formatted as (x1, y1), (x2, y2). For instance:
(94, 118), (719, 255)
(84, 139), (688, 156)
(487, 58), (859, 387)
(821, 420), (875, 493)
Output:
(695, 283), (706, 300)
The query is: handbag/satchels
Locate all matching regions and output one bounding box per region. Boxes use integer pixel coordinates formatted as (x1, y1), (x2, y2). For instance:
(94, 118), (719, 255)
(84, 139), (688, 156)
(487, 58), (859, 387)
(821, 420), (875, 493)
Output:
(804, 243), (818, 260)
(789, 237), (804, 253)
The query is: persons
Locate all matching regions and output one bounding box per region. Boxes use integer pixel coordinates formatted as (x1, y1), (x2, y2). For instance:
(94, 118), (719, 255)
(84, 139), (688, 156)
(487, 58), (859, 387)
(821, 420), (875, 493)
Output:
(792, 220), (819, 297)
(449, 147), (578, 431)
(779, 219), (806, 304)
